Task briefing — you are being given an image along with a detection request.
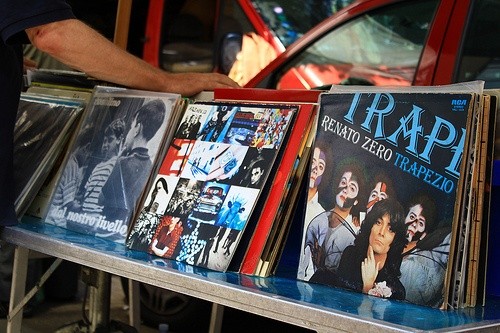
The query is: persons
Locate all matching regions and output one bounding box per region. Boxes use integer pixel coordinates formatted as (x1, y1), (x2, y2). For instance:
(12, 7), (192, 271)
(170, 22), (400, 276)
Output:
(46, 99), (166, 243)
(299, 145), (453, 305)
(0, 0), (240, 227)
(127, 112), (286, 268)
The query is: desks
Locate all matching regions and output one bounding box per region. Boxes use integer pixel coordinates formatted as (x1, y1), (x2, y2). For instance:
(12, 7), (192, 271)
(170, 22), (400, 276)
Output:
(2, 215), (500, 332)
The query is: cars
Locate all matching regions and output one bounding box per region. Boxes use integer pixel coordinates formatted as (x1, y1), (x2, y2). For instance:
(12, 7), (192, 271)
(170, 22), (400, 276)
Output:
(119, 0), (500, 333)
